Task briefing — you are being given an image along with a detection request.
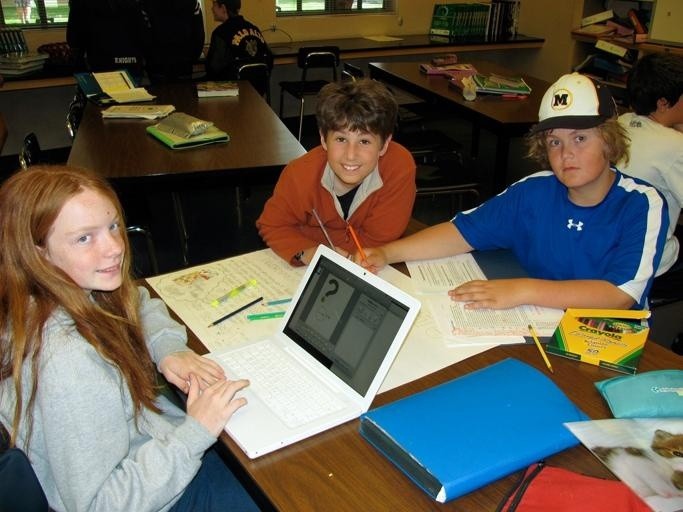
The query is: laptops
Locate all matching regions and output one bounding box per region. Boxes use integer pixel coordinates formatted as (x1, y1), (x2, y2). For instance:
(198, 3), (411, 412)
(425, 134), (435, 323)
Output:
(188, 242), (424, 464)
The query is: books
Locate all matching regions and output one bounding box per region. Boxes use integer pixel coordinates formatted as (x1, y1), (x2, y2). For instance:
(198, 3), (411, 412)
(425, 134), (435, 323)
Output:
(100, 105), (175, 120)
(0, 28), (50, 75)
(570, 9), (649, 83)
(429, 0), (520, 45)
(419, 63), (532, 96)
(146, 126), (231, 150)
(196, 80), (239, 97)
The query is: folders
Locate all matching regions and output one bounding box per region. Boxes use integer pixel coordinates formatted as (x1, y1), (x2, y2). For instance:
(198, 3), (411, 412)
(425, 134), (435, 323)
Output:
(358, 354), (599, 504)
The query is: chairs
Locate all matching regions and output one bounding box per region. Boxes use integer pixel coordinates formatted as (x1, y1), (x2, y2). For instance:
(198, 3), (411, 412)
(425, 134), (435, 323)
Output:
(0, 421), (260, 512)
(403, 149), (480, 216)
(64, 96), (84, 138)
(237, 63), (271, 104)
(279, 45), (340, 143)
(342, 63), (364, 82)
(19, 132), (54, 172)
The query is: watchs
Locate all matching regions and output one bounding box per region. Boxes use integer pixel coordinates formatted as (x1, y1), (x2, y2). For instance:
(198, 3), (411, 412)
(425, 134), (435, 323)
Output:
(295, 250), (304, 264)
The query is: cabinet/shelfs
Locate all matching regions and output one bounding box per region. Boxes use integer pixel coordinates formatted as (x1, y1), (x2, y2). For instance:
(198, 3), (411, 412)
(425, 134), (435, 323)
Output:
(571, 31), (683, 115)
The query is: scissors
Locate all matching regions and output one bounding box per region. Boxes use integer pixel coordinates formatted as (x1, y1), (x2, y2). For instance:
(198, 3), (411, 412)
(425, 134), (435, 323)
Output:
(480, 93), (527, 101)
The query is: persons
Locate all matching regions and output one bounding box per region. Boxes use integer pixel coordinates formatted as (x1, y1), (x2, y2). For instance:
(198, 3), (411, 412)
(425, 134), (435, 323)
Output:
(255, 79), (417, 266)
(611, 53), (683, 299)
(205, 0), (274, 96)
(353, 72), (669, 327)
(0, 164), (270, 512)
(14, 1), (42, 24)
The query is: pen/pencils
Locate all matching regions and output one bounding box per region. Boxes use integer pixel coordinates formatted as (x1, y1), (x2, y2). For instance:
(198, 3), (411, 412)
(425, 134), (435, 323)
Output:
(211, 278), (256, 307)
(347, 224), (373, 274)
(575, 316), (637, 334)
(207, 296), (263, 329)
(248, 312), (285, 319)
(262, 298), (292, 305)
(528, 324), (556, 374)
(312, 208), (334, 251)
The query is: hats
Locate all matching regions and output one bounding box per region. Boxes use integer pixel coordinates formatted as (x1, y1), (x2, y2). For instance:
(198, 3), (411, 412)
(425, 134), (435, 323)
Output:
(529, 70), (617, 136)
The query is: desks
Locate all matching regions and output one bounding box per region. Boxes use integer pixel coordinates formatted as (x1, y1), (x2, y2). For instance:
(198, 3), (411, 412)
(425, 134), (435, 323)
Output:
(370, 58), (552, 185)
(67, 75), (309, 267)
(140, 217), (683, 511)
